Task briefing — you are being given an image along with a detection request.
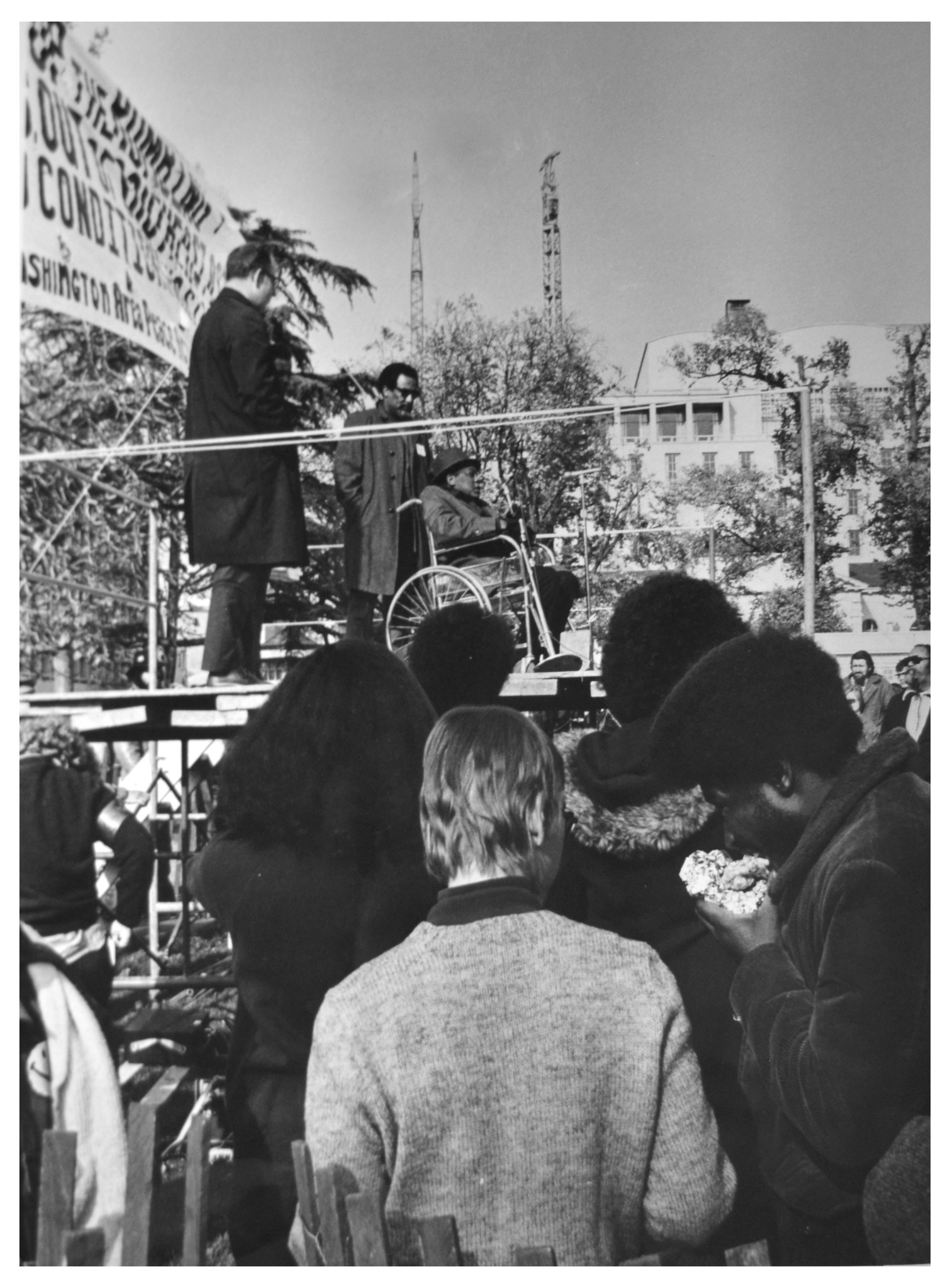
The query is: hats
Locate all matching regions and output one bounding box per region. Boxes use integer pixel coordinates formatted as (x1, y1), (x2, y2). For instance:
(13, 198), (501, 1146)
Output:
(569, 630), (863, 812)
(430, 446), (481, 485)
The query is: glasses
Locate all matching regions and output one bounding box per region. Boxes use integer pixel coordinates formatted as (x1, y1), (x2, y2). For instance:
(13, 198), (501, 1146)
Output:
(395, 387), (419, 399)
(900, 655), (929, 667)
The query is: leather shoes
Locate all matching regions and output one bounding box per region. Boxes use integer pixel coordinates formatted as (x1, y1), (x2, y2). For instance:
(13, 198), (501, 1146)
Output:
(206, 666), (268, 687)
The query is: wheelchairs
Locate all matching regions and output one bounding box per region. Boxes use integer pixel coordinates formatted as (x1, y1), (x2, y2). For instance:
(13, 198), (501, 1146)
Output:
(384, 496), (556, 673)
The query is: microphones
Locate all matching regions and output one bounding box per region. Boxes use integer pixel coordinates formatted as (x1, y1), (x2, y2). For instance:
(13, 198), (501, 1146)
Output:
(491, 495), (498, 504)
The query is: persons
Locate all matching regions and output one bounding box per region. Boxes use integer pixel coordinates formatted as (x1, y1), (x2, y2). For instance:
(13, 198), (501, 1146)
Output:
(183, 243), (309, 686)
(19, 362), (927, 1266)
